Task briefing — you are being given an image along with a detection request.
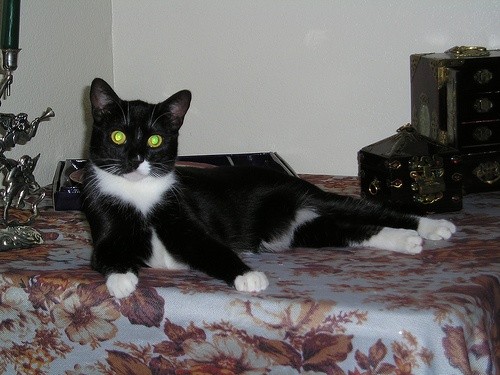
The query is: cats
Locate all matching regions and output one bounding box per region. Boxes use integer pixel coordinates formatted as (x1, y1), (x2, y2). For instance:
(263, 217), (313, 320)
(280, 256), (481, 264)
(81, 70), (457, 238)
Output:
(81, 76), (456, 300)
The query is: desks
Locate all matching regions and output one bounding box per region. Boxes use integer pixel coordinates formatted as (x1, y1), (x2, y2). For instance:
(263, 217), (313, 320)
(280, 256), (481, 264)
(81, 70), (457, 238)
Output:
(0, 165), (500, 375)
